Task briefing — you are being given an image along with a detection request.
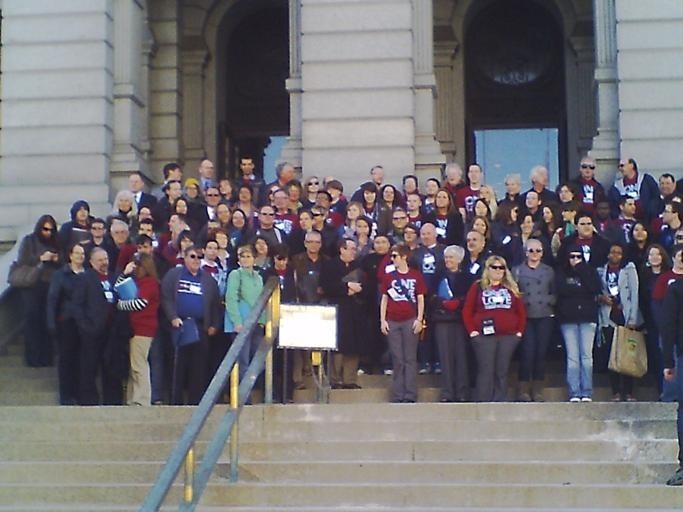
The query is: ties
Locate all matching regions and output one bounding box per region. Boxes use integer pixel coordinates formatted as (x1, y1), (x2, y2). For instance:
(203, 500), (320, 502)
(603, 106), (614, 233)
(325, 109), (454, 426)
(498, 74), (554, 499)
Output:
(202, 182), (210, 190)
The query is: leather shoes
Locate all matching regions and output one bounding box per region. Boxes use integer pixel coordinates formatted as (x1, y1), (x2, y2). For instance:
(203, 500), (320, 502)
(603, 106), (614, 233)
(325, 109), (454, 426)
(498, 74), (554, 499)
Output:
(345, 383), (360, 388)
(331, 384), (342, 389)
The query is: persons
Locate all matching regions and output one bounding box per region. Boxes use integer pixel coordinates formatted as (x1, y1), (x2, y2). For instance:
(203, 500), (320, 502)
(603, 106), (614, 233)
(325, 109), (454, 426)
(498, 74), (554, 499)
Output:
(379, 244), (427, 403)
(262, 161), (295, 206)
(401, 174), (426, 210)
(462, 230), (493, 290)
(128, 172), (158, 217)
(387, 206), (421, 245)
(164, 218), (197, 275)
(518, 189), (543, 224)
(252, 236), (274, 268)
(596, 240), (644, 402)
(325, 179), (347, 214)
(290, 207), (326, 253)
(537, 201), (563, 242)
(168, 197), (200, 243)
(219, 177), (239, 207)
(199, 239), (227, 364)
(638, 243), (670, 400)
(431, 245), (475, 402)
(479, 184), (499, 220)
(402, 224), (422, 268)
(158, 214), (188, 255)
(458, 198), (492, 242)
(609, 157), (661, 227)
(520, 164), (557, 203)
(267, 183), (280, 212)
(570, 156), (606, 218)
(673, 228), (683, 245)
(197, 185), (222, 230)
(70, 246), (119, 405)
(272, 187), (300, 236)
(59, 200), (96, 262)
(411, 222), (448, 375)
(337, 201), (366, 242)
(240, 205), (288, 245)
(84, 218), (117, 270)
(226, 207), (253, 251)
(161, 244), (222, 405)
(422, 178), (441, 215)
(655, 200), (683, 255)
(319, 237), (367, 389)
(424, 188), (462, 247)
(356, 233), (394, 376)
(262, 243), (298, 404)
(455, 163), (484, 224)
(295, 231), (333, 389)
(284, 178), (307, 212)
(557, 209), (608, 273)
(315, 189), (345, 229)
(406, 193), (426, 230)
(470, 213), (497, 255)
(652, 244), (683, 304)
(230, 185), (259, 228)
(592, 197), (626, 243)
(137, 217), (157, 238)
(461, 255), (528, 402)
(310, 204), (339, 258)
(114, 251), (161, 406)
(555, 246), (603, 402)
(613, 194), (640, 242)
(441, 163), (468, 200)
(497, 174), (522, 206)
(304, 175), (321, 209)
(490, 200), (524, 270)
(196, 157), (219, 192)
(207, 227), (237, 273)
(223, 244), (267, 405)
(104, 188), (137, 233)
(508, 211), (555, 269)
(109, 219), (137, 276)
(17, 214), (66, 368)
(131, 203), (156, 234)
(350, 215), (374, 267)
(150, 163), (183, 202)
(135, 233), (171, 405)
(557, 180), (578, 204)
(233, 155), (266, 207)
(551, 199), (597, 257)
(358, 182), (391, 237)
(644, 171), (682, 226)
(510, 238), (559, 403)
(350, 165), (384, 203)
(157, 178), (183, 231)
(183, 177), (206, 211)
(199, 201), (231, 244)
(45, 242), (88, 406)
(380, 184), (405, 236)
(662, 250), (683, 486)
(625, 219), (654, 275)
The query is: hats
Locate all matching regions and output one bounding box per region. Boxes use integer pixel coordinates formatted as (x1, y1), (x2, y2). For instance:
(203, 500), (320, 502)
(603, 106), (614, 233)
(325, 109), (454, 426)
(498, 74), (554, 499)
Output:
(183, 178), (201, 190)
(569, 244), (584, 253)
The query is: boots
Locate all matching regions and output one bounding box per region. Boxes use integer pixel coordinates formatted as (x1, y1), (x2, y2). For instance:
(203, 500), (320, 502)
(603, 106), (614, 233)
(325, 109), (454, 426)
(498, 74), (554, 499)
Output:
(517, 381), (532, 402)
(531, 379), (546, 403)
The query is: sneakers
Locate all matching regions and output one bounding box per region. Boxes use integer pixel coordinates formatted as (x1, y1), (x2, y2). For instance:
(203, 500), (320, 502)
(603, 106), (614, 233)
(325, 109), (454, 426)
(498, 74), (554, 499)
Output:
(434, 362), (440, 373)
(667, 468), (682, 487)
(626, 394), (638, 402)
(581, 396), (592, 403)
(382, 363), (392, 376)
(610, 394), (621, 400)
(569, 396), (580, 403)
(419, 364), (433, 374)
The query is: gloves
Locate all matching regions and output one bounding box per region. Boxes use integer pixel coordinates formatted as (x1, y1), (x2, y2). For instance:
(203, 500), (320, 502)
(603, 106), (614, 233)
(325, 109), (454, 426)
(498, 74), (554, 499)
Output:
(442, 299), (460, 312)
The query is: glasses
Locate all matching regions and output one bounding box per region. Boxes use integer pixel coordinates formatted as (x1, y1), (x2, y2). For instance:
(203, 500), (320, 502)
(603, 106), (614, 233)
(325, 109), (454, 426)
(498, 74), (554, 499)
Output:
(206, 193), (221, 197)
(579, 163), (596, 170)
(568, 254), (583, 259)
(579, 221), (592, 226)
(259, 212), (275, 218)
(676, 234), (682, 239)
(618, 163), (634, 168)
(391, 254), (401, 259)
(489, 264), (507, 270)
(311, 212), (324, 218)
(42, 226), (54, 233)
(392, 215), (408, 220)
(186, 253), (203, 260)
(308, 180), (321, 186)
(90, 226), (105, 232)
(525, 247), (545, 254)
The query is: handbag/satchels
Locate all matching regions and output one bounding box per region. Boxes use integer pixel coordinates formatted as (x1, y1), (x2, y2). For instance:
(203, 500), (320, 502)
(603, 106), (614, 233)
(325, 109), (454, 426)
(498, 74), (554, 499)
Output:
(8, 236), (41, 287)
(607, 322), (648, 380)
(393, 271), (430, 343)
(595, 296), (615, 347)
